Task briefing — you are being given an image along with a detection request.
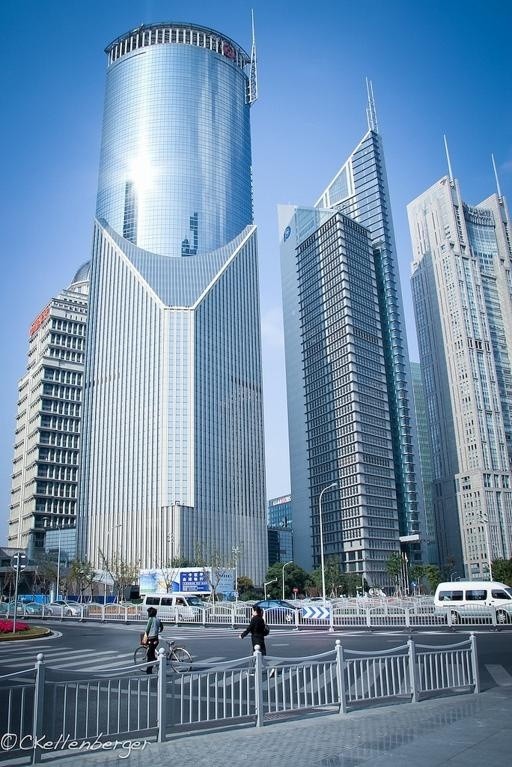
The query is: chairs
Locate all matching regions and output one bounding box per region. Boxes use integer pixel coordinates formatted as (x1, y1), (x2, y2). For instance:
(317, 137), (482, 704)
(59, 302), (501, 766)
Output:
(269, 669), (275, 679)
(245, 672), (254, 677)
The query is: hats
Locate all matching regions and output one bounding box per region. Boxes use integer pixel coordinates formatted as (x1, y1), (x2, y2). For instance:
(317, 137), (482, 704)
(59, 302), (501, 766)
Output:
(0, 599), (88, 617)
(251, 600), (301, 621)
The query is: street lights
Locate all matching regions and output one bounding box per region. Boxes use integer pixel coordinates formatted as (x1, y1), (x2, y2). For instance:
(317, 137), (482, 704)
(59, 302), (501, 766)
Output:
(263, 623), (269, 637)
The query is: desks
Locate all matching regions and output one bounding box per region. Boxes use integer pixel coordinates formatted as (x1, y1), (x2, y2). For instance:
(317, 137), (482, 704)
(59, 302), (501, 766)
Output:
(283, 560), (293, 600)
(42, 516), (61, 600)
(319, 483), (338, 601)
(479, 511), (492, 581)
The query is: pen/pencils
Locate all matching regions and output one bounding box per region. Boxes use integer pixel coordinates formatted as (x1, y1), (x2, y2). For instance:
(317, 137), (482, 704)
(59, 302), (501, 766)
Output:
(140, 593), (209, 620)
(433, 581), (512, 624)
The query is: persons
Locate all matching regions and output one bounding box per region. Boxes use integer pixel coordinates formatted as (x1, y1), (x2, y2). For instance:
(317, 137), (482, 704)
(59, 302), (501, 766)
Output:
(239, 605), (266, 656)
(143, 607), (163, 674)
